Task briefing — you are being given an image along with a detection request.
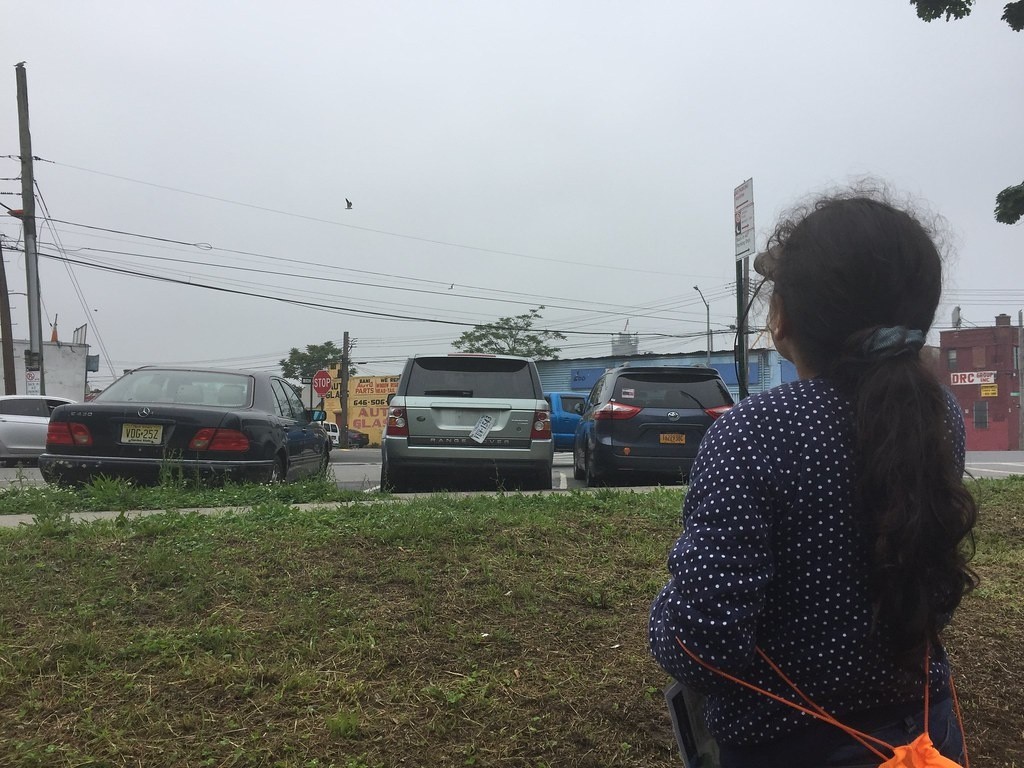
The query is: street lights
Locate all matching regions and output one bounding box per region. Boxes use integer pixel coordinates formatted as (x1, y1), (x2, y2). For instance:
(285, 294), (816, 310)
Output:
(693, 285), (712, 366)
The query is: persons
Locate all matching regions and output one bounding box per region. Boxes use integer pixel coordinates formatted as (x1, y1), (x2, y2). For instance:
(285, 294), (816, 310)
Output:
(648, 197), (980, 768)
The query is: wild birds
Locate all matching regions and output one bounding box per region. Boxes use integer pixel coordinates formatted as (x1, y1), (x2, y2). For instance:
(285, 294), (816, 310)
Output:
(345, 197), (353, 210)
(448, 283), (455, 290)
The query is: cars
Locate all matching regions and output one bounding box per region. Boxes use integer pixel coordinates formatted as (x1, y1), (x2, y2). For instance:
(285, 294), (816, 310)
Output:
(39, 361), (334, 493)
(315, 421), (340, 446)
(0, 395), (77, 466)
(345, 429), (370, 449)
(541, 391), (591, 451)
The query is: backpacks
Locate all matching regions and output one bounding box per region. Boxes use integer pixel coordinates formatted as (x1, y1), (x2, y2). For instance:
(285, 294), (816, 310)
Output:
(672, 624), (971, 767)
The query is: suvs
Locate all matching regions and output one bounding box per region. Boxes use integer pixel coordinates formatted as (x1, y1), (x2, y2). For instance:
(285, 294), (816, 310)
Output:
(572, 361), (740, 486)
(381, 352), (556, 494)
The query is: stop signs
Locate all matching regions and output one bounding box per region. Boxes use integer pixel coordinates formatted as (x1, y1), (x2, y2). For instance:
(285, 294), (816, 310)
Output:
(312, 370), (332, 395)
